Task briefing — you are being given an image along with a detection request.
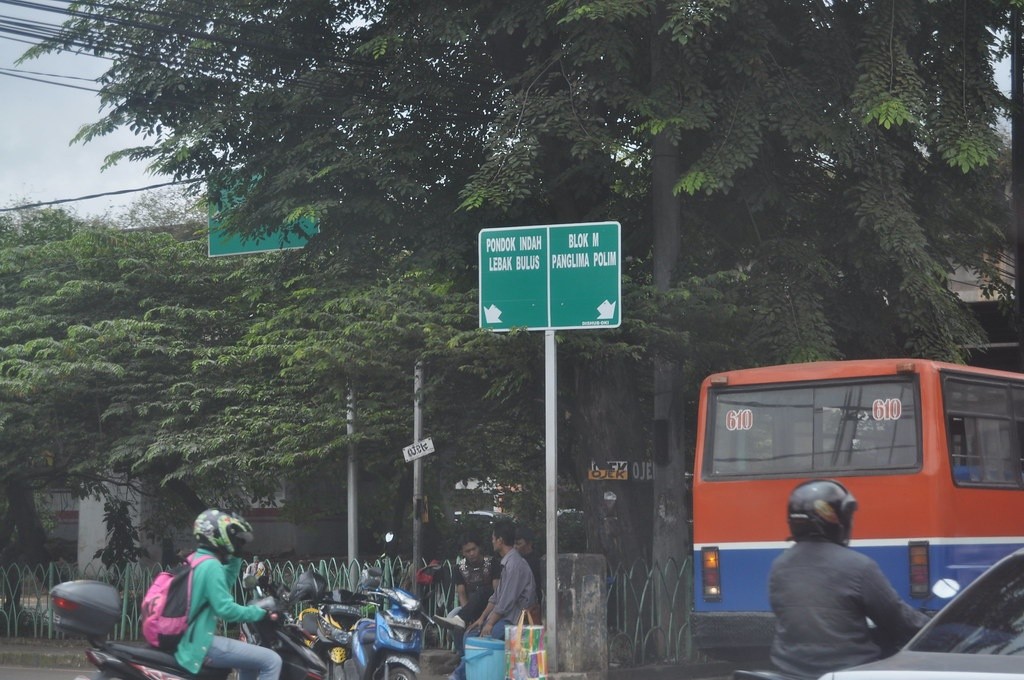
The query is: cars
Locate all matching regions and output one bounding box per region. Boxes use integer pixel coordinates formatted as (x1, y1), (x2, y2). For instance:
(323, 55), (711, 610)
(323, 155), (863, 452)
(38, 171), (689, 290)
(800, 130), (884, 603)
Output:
(816, 547), (1024, 680)
(454, 510), (508, 522)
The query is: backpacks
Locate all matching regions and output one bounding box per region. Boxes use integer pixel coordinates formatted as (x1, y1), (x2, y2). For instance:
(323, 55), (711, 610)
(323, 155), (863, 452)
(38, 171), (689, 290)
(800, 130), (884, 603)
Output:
(141, 553), (217, 651)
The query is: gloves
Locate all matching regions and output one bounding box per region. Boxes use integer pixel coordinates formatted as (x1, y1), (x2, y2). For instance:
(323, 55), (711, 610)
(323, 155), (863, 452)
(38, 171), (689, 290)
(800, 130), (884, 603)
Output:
(257, 596), (282, 614)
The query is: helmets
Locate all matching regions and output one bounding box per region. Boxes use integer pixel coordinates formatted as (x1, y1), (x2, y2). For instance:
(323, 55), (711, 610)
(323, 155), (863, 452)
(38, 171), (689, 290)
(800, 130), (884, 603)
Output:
(786, 479), (859, 546)
(288, 570), (327, 605)
(194, 507), (252, 554)
(242, 561), (274, 595)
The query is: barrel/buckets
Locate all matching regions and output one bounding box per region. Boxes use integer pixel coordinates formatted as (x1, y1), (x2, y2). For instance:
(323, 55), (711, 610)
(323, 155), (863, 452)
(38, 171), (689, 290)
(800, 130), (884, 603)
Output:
(461, 635), (505, 680)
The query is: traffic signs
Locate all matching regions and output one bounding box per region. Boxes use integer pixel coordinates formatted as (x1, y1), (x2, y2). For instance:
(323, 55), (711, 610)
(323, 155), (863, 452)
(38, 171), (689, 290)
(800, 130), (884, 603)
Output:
(478, 220), (622, 332)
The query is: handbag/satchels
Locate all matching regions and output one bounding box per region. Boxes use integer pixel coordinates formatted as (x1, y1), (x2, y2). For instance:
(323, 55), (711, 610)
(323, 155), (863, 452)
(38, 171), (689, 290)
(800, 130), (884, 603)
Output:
(504, 608), (547, 680)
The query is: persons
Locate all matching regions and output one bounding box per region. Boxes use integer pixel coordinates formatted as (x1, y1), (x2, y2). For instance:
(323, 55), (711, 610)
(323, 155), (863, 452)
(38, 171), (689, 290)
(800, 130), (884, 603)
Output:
(246, 556), (265, 575)
(173, 507), (282, 680)
(433, 521), (541, 680)
(768, 479), (932, 680)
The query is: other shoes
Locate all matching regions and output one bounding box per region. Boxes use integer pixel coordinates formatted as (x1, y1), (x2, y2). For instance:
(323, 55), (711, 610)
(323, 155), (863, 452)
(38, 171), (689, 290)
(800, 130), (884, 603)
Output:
(433, 614), (465, 632)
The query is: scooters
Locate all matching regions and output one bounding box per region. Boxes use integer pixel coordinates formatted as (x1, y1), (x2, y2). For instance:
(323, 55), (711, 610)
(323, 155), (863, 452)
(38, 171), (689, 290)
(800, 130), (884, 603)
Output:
(240, 529), (427, 680)
(46, 572), (328, 680)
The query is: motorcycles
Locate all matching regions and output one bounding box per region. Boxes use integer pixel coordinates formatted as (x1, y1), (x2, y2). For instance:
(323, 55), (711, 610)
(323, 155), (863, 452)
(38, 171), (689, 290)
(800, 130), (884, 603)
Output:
(732, 578), (958, 680)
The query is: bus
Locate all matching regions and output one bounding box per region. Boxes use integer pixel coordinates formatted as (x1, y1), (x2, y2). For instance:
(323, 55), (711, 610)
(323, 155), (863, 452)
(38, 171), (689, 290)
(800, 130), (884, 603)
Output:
(694, 359), (1024, 666)
